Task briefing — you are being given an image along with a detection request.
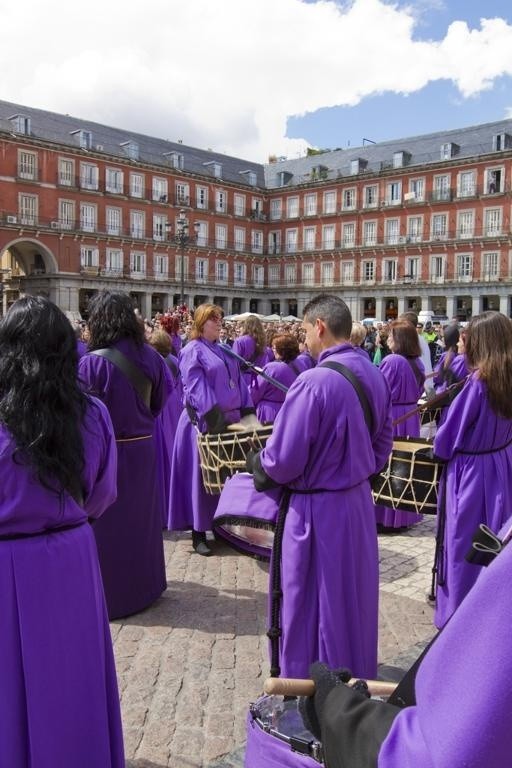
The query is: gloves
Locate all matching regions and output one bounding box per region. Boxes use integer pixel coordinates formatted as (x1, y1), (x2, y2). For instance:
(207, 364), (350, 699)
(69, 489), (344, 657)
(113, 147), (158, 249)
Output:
(298, 660), (371, 742)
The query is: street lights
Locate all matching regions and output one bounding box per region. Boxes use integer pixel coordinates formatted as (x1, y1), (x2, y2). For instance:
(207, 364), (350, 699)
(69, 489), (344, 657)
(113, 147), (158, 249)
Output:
(161, 206), (202, 304)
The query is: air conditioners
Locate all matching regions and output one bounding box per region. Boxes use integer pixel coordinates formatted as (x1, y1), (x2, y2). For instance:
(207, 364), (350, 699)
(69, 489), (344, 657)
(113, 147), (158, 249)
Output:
(50, 221), (58, 229)
(7, 215), (17, 224)
(397, 236), (406, 245)
(381, 200), (389, 207)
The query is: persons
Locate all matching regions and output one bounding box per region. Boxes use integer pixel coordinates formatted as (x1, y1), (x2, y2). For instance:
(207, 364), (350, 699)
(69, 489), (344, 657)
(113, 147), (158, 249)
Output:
(433, 311), (511, 631)
(302, 517), (511, 767)
(0, 296), (126, 766)
(264, 293), (397, 678)
(353, 311), (473, 533)
(78, 290), (316, 622)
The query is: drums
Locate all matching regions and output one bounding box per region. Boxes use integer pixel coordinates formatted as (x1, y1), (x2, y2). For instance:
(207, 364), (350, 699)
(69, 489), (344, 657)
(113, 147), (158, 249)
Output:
(224, 415), (264, 432)
(194, 423), (276, 496)
(243, 685), (388, 768)
(370, 434), (440, 517)
(414, 396), (441, 425)
(211, 469), (280, 565)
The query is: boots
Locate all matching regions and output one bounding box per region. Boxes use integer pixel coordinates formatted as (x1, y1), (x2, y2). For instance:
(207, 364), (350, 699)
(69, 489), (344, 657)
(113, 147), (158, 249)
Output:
(192, 529), (212, 555)
